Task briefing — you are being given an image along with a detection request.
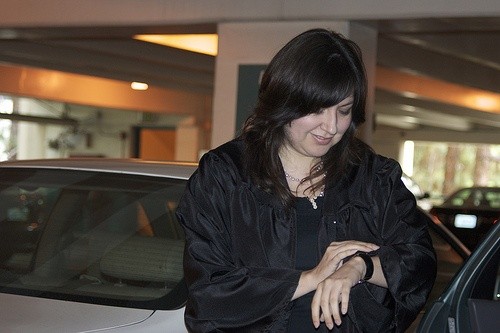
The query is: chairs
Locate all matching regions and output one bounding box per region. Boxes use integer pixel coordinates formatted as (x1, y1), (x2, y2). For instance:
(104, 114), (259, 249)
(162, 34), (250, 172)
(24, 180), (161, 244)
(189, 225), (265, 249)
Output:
(59, 231), (186, 289)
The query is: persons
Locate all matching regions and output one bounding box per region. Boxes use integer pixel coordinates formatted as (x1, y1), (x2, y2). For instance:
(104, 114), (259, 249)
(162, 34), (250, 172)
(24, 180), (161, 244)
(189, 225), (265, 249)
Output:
(49, 190), (113, 273)
(174, 28), (438, 333)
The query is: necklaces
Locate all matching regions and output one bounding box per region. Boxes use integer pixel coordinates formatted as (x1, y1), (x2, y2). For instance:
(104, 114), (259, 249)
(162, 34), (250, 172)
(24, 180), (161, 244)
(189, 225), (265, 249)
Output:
(285, 161), (328, 209)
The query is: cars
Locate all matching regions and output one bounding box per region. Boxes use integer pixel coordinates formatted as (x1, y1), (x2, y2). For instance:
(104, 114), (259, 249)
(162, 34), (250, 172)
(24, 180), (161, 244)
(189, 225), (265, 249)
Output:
(430, 186), (500, 255)
(411, 210), (500, 333)
(0, 157), (200, 333)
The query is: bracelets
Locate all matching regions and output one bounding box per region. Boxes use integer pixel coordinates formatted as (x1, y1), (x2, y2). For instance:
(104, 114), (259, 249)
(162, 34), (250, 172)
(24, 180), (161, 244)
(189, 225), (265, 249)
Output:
(352, 253), (374, 284)
